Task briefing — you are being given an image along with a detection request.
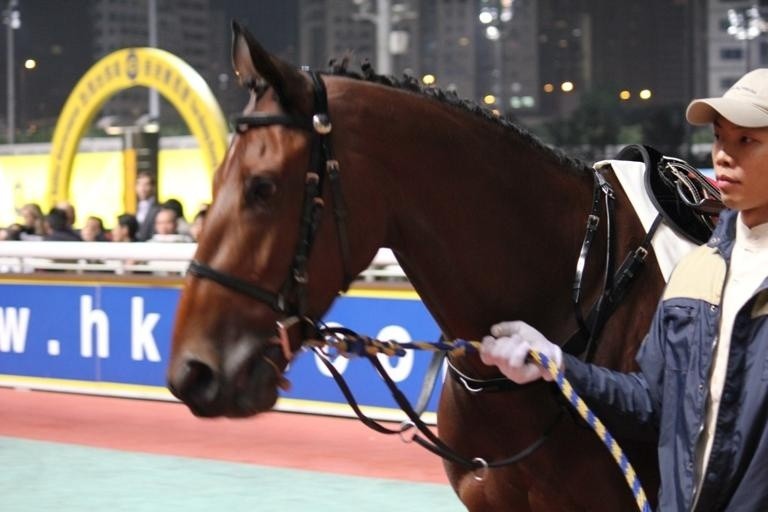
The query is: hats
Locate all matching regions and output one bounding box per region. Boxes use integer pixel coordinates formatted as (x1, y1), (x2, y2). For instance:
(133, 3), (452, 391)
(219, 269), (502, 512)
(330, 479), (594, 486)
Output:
(684, 64), (768, 131)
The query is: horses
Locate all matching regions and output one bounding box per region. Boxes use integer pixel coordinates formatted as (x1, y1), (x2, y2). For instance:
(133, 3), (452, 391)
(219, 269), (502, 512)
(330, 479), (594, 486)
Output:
(162, 15), (723, 512)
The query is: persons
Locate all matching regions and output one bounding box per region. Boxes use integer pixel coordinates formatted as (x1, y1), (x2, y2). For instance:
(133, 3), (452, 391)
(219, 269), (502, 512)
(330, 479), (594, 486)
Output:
(481, 67), (768, 512)
(0, 173), (212, 275)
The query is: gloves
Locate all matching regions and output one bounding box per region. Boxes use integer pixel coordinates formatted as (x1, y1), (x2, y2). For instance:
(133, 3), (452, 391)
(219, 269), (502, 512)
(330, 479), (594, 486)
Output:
(477, 317), (565, 388)
